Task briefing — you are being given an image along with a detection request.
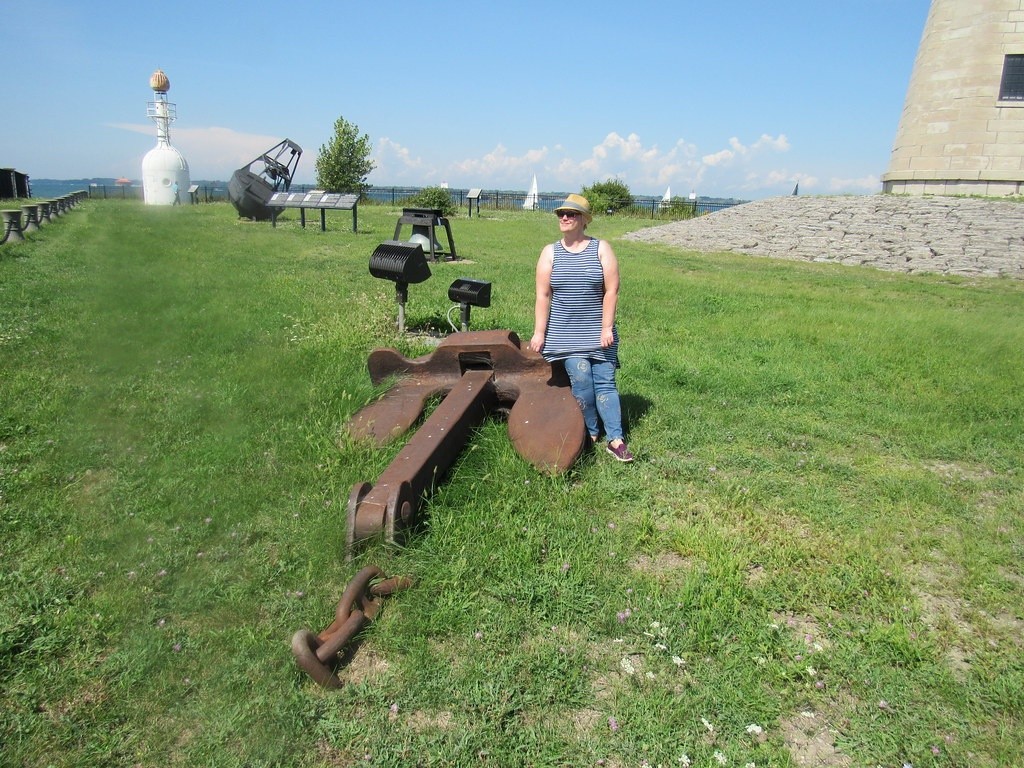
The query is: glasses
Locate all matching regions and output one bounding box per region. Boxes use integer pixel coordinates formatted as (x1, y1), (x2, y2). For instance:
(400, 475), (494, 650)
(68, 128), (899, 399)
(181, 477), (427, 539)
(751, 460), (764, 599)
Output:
(558, 211), (582, 218)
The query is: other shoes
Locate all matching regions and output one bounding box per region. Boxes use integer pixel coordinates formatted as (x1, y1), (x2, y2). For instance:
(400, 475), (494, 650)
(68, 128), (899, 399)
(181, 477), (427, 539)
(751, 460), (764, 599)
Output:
(585, 436), (599, 450)
(606, 442), (633, 462)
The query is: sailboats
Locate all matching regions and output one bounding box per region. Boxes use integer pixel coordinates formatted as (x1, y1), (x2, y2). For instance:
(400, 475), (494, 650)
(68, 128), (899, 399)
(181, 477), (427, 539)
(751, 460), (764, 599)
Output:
(521, 173), (540, 210)
(658, 186), (674, 208)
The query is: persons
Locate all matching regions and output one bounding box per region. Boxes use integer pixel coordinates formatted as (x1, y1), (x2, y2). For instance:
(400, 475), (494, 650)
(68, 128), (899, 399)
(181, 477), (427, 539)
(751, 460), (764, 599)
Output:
(530, 193), (635, 461)
(171, 181), (181, 206)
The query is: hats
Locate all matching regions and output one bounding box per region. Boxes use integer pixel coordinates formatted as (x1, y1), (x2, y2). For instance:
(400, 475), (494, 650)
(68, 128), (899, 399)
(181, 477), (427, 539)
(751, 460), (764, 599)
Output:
(553, 194), (592, 224)
